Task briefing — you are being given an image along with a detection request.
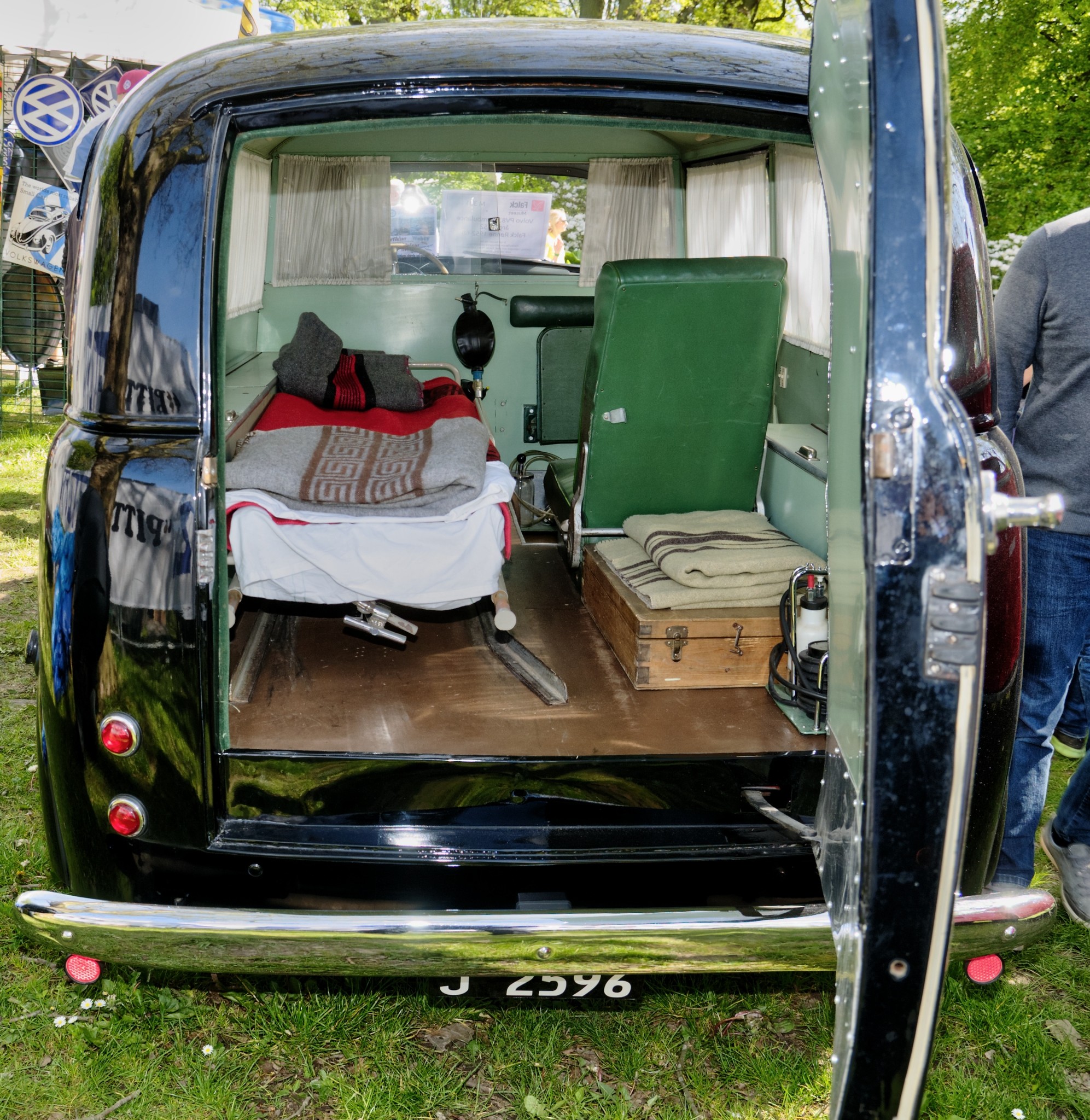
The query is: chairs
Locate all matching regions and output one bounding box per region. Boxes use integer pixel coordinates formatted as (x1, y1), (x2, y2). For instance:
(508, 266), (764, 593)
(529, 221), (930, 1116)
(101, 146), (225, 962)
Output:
(546, 258), (788, 569)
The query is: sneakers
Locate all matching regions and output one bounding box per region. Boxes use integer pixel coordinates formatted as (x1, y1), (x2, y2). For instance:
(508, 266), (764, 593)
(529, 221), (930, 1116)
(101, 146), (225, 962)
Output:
(980, 883), (1026, 895)
(1039, 817), (1090, 928)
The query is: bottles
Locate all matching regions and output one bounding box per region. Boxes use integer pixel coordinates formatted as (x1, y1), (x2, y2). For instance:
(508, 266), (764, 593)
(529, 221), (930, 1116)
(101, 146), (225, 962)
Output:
(793, 575), (828, 665)
(797, 640), (828, 719)
(510, 453), (534, 524)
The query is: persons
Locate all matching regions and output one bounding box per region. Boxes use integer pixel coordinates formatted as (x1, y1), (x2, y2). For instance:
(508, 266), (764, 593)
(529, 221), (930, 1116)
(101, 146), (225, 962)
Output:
(986, 205), (1090, 929)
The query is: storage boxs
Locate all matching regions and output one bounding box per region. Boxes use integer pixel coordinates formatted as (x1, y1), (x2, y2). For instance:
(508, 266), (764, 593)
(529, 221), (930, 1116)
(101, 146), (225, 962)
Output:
(583, 543), (794, 690)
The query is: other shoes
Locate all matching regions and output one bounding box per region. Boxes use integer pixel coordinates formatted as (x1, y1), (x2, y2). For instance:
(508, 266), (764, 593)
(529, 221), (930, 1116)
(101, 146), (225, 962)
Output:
(1051, 730), (1086, 758)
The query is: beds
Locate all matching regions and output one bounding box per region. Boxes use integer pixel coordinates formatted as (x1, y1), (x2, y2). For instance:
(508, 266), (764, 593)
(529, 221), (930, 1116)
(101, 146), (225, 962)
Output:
(226, 362), (569, 709)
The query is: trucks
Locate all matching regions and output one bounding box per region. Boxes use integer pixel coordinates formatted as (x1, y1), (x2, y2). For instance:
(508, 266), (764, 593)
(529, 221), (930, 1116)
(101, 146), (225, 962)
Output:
(14, 15), (1060, 1115)
